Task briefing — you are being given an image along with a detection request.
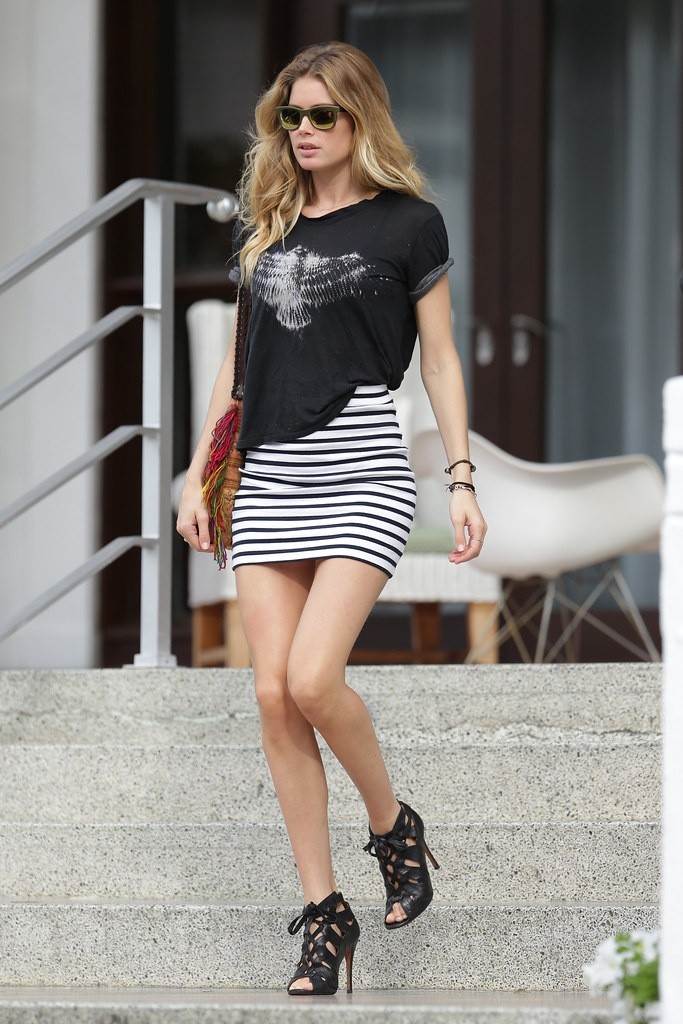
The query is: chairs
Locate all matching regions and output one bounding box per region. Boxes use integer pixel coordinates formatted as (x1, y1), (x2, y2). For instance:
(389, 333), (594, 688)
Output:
(410, 428), (665, 663)
(171, 301), (500, 668)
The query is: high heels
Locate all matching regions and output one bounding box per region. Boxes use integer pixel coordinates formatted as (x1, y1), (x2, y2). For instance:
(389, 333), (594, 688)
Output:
(363, 798), (440, 930)
(287, 891), (360, 996)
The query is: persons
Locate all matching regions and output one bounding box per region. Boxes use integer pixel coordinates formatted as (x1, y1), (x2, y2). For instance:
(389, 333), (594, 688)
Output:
(174, 41), (487, 994)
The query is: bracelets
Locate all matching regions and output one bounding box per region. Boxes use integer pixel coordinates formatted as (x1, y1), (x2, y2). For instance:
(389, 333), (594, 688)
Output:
(446, 482), (477, 497)
(445, 459), (477, 475)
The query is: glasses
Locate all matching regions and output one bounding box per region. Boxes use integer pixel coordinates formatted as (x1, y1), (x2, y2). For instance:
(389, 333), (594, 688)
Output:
(275, 105), (347, 131)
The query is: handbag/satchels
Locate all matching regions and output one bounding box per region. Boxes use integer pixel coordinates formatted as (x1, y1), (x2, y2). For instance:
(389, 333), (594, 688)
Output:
(200, 399), (242, 571)
